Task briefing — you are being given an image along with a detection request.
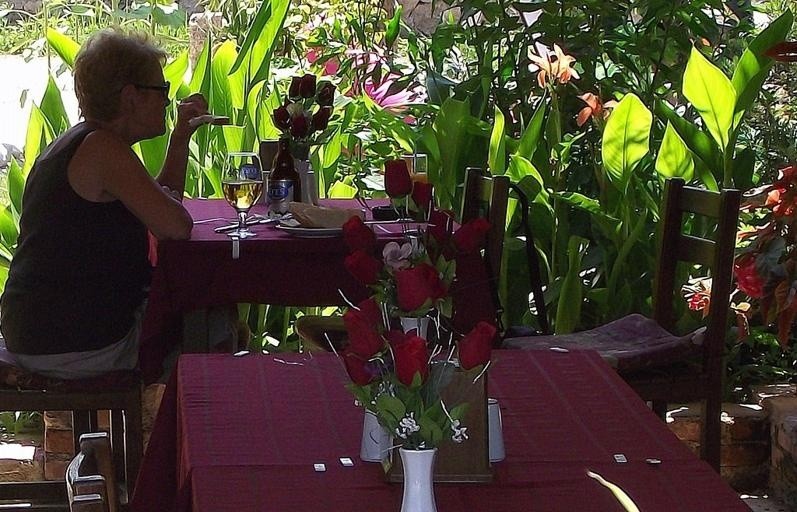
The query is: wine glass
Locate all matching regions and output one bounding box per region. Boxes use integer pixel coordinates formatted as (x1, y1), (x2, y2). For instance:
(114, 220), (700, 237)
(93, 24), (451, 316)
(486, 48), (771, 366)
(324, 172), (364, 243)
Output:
(222, 153), (262, 238)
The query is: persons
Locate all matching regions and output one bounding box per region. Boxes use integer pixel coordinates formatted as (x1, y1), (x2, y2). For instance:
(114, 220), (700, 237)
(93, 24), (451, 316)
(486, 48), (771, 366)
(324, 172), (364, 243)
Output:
(0, 28), (253, 512)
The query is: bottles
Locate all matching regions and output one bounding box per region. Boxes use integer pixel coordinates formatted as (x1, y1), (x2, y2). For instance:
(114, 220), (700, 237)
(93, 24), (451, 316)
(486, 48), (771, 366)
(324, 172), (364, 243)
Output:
(267, 137), (301, 213)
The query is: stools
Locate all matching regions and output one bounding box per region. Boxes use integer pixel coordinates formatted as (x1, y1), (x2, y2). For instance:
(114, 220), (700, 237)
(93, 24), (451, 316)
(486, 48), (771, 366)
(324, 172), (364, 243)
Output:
(2, 360), (146, 511)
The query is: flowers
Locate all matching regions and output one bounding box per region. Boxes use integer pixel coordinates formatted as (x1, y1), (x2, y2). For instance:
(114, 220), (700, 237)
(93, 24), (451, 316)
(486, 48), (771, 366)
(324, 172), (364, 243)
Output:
(273, 75), (337, 161)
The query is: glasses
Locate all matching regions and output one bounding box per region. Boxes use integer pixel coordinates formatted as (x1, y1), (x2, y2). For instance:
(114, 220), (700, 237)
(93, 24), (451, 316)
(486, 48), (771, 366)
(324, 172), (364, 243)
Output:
(137, 81), (170, 97)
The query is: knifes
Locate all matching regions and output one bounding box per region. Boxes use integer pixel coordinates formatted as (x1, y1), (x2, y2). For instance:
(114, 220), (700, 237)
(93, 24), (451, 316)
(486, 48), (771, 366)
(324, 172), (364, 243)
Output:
(213, 216), (295, 234)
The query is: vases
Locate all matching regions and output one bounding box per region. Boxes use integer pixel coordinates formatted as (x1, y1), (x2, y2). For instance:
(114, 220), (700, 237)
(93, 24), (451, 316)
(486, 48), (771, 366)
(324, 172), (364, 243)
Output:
(286, 156), (313, 206)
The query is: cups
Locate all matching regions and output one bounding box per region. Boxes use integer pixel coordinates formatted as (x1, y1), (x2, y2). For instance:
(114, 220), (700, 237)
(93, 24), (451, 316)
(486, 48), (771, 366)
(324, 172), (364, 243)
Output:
(476, 399), (507, 463)
